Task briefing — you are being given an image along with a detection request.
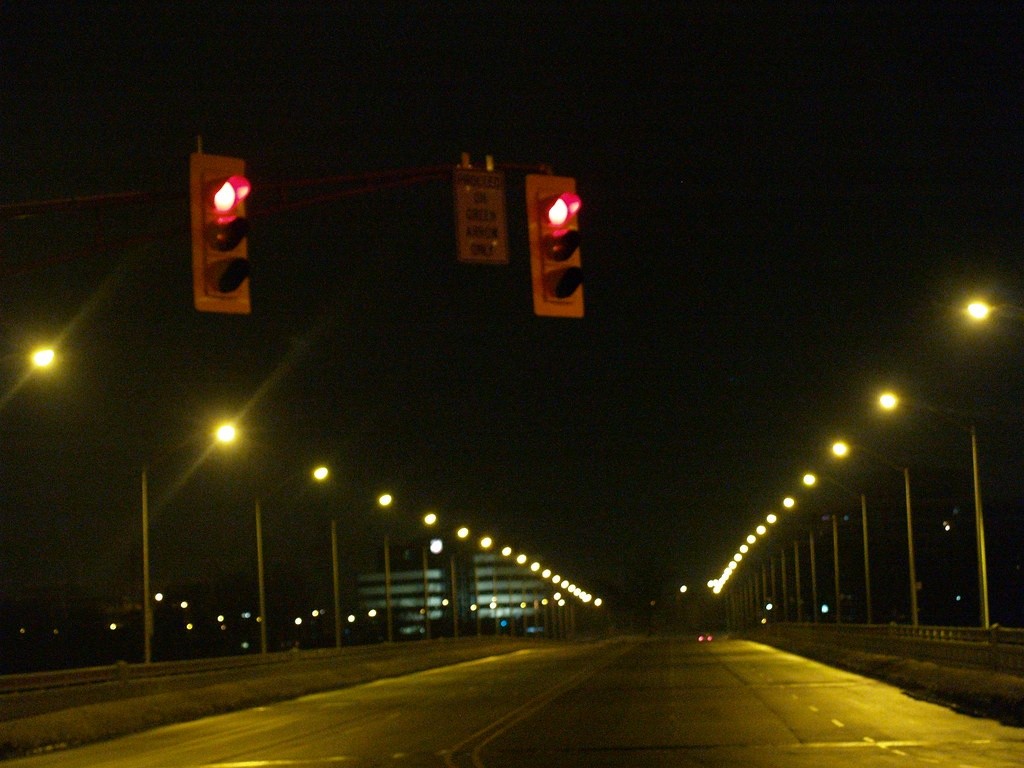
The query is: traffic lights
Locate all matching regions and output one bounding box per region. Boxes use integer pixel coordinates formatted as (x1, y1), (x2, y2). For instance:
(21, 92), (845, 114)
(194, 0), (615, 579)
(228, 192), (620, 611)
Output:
(188, 152), (255, 316)
(526, 171), (588, 318)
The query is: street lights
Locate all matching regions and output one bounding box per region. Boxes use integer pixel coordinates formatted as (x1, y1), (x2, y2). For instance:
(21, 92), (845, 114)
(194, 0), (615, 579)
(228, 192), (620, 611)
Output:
(330, 493), (393, 650)
(878, 392), (991, 627)
(385, 514), (437, 643)
(450, 537), (492, 639)
(422, 527), (468, 641)
(832, 440), (918, 629)
(472, 545), (603, 638)
(254, 466), (331, 653)
(782, 496), (819, 625)
(140, 426), (235, 662)
(709, 514), (804, 625)
(802, 472), (874, 625)
